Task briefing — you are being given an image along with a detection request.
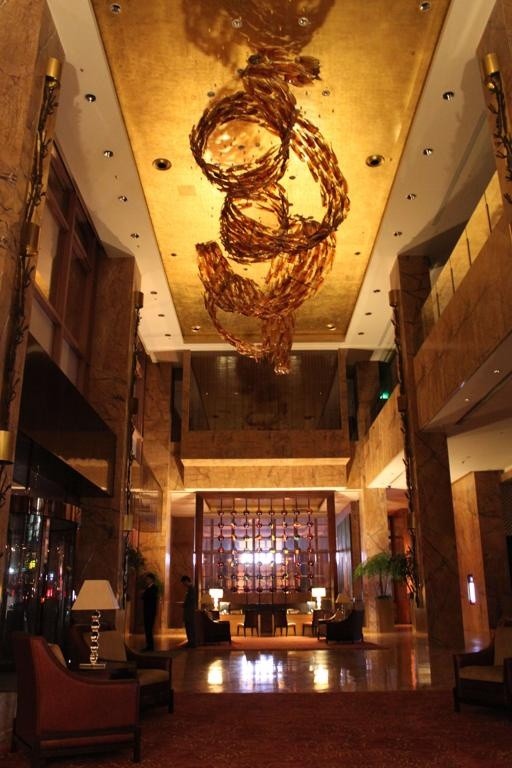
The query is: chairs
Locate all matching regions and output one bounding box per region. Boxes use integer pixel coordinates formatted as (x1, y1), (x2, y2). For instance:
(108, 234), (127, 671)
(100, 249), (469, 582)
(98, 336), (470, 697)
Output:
(236, 608), (331, 636)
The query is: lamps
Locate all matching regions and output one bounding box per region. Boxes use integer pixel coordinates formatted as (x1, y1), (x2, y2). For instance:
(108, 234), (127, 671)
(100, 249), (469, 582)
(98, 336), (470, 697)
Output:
(208, 588), (224, 611)
(334, 592), (351, 609)
(312, 586), (327, 608)
(72, 579), (120, 669)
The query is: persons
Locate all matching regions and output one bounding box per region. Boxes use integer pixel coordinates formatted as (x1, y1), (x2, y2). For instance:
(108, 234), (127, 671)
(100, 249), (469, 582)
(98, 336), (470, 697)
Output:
(182, 574), (197, 648)
(141, 572), (159, 652)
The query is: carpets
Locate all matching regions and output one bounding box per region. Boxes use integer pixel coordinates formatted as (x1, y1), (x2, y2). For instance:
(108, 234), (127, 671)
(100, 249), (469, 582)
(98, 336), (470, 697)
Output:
(174, 633), (389, 650)
(0, 689), (512, 767)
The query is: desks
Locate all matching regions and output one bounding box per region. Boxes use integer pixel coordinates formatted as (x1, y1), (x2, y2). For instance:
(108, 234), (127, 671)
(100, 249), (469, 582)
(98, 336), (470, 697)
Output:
(75, 660), (137, 679)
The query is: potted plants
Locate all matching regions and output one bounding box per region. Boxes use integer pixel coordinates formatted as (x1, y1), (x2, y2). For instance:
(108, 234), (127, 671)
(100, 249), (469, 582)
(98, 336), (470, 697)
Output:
(355, 552), (411, 632)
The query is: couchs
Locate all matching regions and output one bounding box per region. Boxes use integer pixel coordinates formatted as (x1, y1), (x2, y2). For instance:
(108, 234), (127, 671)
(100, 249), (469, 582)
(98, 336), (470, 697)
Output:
(11, 633), (141, 767)
(452, 611), (511, 715)
(325, 608), (365, 644)
(69, 622), (174, 718)
(193, 610), (231, 642)
